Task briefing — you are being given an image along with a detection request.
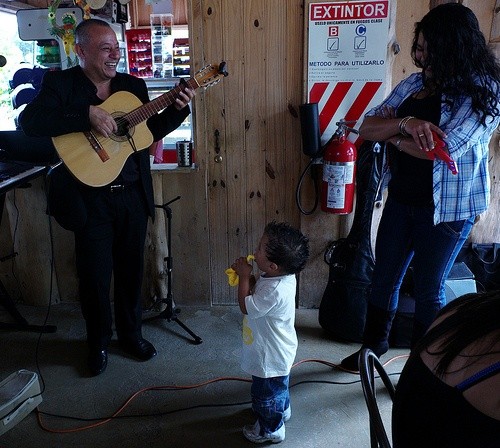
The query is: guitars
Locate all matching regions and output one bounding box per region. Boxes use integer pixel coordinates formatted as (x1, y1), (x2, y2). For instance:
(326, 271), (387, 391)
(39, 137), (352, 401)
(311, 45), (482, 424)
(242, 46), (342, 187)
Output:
(50, 61), (229, 188)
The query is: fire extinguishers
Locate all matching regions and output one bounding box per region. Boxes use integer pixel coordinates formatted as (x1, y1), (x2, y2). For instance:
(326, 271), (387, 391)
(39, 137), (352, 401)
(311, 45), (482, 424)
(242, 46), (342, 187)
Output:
(320, 119), (360, 216)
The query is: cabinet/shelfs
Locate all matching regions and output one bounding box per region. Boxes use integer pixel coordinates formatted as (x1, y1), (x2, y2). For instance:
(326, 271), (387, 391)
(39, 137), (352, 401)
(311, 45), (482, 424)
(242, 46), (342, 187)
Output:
(144, 78), (193, 164)
(126, 13), (190, 79)
(17, 8), (127, 74)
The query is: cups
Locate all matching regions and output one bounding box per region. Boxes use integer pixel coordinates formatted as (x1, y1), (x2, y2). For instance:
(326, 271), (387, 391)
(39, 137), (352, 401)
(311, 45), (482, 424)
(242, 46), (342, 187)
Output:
(176, 141), (192, 166)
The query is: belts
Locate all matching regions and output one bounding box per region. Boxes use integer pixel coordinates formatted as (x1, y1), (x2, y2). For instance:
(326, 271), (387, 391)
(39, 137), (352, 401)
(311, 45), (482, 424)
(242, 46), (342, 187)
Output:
(99, 183), (133, 193)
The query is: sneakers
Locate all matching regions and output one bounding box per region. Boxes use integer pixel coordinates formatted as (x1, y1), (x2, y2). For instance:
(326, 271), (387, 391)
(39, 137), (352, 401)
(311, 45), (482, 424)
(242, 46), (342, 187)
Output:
(282, 405), (292, 422)
(243, 419), (286, 444)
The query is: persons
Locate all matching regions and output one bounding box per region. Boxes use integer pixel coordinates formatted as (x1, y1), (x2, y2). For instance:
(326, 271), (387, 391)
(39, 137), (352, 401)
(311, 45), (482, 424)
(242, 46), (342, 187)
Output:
(341, 3), (500, 371)
(19, 19), (195, 373)
(392, 288), (500, 448)
(232, 222), (309, 442)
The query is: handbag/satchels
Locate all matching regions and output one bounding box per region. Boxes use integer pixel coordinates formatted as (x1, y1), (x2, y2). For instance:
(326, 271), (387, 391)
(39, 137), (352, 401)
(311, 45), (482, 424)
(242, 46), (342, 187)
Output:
(458, 242), (498, 291)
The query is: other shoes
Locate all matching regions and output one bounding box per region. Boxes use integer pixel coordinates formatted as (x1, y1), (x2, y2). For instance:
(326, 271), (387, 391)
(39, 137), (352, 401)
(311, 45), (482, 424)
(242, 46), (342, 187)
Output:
(341, 347), (381, 373)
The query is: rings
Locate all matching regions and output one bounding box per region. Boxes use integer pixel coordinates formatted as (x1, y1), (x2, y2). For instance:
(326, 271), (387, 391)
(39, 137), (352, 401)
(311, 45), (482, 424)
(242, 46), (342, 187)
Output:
(419, 134), (424, 136)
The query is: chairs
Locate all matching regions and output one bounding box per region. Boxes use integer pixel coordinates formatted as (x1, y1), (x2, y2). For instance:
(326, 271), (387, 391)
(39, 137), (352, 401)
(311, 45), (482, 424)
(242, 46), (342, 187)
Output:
(358, 348), (396, 448)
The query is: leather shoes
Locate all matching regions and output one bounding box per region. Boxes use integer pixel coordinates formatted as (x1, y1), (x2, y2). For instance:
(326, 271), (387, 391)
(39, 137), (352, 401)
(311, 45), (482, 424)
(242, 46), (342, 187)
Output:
(120, 338), (157, 362)
(80, 345), (108, 378)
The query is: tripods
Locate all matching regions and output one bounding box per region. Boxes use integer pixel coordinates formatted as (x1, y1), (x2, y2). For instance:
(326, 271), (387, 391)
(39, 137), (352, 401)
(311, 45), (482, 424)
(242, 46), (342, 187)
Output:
(140, 195), (203, 346)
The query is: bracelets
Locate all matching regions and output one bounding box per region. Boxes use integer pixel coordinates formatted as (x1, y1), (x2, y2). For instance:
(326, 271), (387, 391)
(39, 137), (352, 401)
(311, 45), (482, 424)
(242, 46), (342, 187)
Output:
(397, 136), (405, 151)
(399, 116), (416, 137)
